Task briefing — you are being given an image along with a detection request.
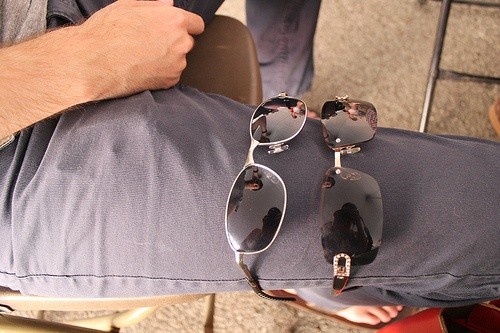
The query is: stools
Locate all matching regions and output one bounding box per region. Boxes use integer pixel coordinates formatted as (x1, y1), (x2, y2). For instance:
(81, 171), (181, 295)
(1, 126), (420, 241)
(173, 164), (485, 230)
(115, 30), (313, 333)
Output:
(0, 14), (263, 331)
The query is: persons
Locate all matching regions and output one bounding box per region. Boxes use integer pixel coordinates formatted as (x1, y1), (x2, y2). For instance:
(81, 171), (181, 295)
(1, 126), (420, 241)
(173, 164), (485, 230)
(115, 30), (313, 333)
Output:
(323, 98), (376, 132)
(1, 0), (500, 326)
(252, 94), (303, 140)
(228, 167), (281, 250)
(323, 202), (373, 262)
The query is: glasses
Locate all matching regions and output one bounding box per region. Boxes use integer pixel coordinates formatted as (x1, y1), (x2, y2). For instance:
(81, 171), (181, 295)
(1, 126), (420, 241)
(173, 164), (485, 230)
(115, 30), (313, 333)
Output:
(318, 94), (387, 296)
(225, 91), (308, 302)
